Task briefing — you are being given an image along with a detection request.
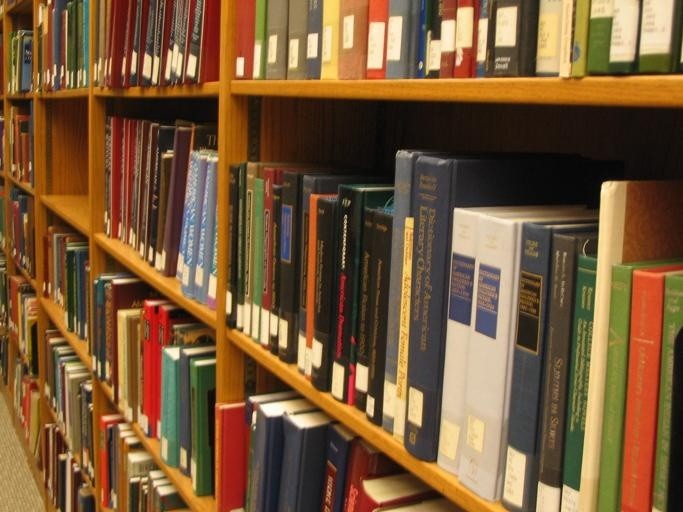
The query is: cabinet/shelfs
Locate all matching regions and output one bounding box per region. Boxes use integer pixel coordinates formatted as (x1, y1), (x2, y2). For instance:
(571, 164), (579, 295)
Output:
(0, 1), (683, 512)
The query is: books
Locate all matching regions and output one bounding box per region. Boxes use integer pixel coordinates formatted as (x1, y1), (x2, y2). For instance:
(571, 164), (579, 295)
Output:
(39, 328), (190, 511)
(1, 185), (37, 279)
(86, 271), (216, 498)
(229, 0), (683, 80)
(37, 222), (92, 340)
(224, 143), (682, 511)
(33, 2), (221, 90)
(102, 110), (221, 311)
(0, 254), (42, 377)
(7, 28), (34, 95)
(1, 330), (45, 473)
(1, 100), (35, 188)
